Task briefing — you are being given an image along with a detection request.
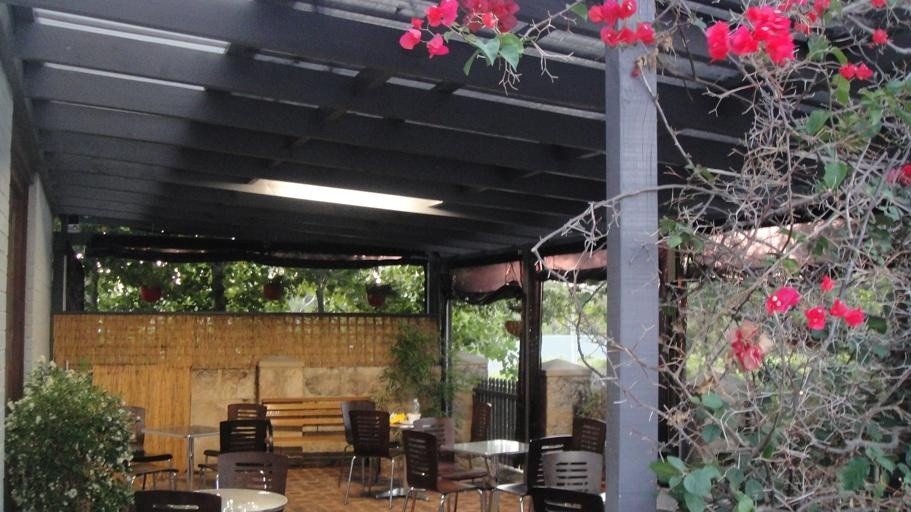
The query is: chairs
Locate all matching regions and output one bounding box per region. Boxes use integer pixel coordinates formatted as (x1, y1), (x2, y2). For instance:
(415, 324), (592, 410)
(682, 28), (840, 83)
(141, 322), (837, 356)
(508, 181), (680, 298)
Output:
(335, 394), (608, 510)
(111, 393), (291, 511)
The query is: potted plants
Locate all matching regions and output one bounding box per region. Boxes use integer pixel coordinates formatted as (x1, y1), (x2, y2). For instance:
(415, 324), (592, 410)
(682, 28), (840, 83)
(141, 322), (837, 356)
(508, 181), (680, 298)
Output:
(263, 278), (289, 298)
(138, 279), (167, 300)
(366, 282), (397, 306)
